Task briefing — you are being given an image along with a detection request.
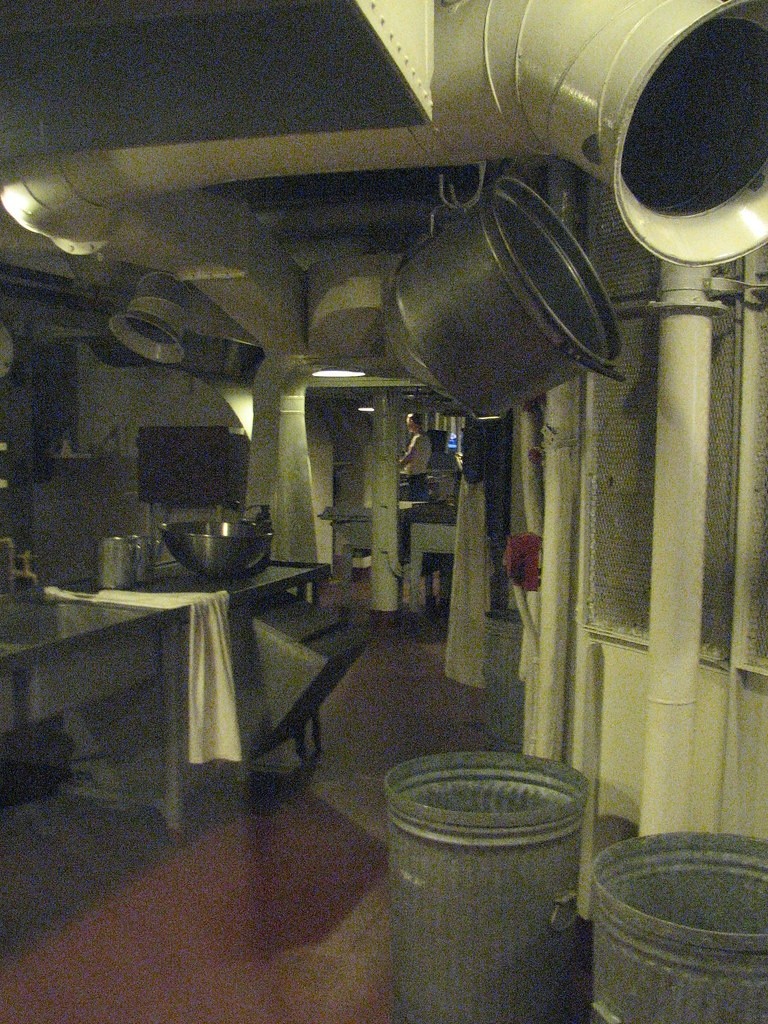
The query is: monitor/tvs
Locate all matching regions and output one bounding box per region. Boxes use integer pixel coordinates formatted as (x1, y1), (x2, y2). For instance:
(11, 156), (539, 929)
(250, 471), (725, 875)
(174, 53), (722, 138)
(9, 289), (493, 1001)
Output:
(137, 427), (232, 507)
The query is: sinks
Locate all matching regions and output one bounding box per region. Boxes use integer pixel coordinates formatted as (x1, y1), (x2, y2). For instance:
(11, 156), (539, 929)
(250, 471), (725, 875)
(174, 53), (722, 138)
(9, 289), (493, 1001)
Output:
(2, 597), (164, 733)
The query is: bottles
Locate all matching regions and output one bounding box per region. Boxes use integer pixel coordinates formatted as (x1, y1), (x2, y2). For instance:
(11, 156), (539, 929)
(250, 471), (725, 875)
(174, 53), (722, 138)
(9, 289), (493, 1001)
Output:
(98, 533), (141, 591)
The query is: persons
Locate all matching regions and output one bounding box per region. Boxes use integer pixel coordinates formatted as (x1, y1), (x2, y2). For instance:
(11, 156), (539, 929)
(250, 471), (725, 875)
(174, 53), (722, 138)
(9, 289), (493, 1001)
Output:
(399, 413), (460, 501)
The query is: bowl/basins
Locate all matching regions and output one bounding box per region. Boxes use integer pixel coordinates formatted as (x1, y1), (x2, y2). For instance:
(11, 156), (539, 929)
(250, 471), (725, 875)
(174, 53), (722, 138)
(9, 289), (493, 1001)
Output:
(157, 521), (275, 579)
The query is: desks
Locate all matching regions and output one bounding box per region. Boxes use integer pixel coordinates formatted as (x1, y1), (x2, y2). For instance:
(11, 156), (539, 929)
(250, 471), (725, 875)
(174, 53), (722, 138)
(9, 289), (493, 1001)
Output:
(345, 521), (458, 613)
(0, 559), (333, 848)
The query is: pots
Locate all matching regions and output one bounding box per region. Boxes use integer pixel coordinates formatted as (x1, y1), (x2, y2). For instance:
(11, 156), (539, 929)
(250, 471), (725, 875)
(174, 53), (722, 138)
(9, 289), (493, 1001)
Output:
(383, 174), (627, 418)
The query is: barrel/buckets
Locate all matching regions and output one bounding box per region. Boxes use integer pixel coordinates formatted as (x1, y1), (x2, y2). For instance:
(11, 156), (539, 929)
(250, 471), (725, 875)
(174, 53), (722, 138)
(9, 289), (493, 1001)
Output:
(481, 609), (524, 687)
(592, 831), (768, 1024)
(383, 749), (590, 1024)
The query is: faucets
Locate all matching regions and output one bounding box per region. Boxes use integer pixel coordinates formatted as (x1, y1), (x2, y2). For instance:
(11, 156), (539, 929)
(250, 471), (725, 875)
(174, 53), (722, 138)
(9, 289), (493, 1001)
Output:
(19, 550), (41, 587)
